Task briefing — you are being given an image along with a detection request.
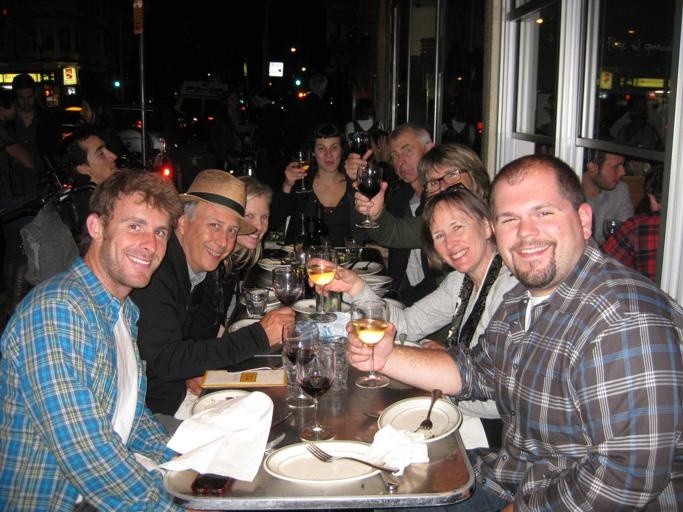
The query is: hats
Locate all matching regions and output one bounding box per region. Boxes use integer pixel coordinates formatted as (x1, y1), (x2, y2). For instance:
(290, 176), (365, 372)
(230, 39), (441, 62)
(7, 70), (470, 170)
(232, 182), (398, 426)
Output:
(178, 169), (257, 235)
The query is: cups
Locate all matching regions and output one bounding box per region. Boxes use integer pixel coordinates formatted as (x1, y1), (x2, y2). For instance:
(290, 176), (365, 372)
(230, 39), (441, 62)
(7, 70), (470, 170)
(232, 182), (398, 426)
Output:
(244, 265), (303, 320)
(603, 219), (623, 241)
(344, 239), (365, 261)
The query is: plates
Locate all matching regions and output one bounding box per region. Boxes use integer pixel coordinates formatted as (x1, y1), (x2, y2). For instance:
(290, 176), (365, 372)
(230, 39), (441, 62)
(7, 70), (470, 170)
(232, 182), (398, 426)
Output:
(256, 258), (292, 274)
(190, 384), (249, 417)
(263, 389), (463, 487)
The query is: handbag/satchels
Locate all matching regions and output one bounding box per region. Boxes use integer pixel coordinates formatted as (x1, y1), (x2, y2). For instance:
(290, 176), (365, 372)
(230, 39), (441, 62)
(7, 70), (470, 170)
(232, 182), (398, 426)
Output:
(0, 148), (40, 208)
(20, 202), (80, 286)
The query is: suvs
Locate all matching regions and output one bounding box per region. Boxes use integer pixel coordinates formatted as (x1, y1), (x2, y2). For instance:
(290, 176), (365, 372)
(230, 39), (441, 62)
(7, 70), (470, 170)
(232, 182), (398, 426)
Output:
(104, 107), (167, 155)
(51, 97), (96, 135)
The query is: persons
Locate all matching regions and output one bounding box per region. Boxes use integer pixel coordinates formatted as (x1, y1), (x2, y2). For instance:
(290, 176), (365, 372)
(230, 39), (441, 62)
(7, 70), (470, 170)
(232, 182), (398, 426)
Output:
(0, 73), (682, 512)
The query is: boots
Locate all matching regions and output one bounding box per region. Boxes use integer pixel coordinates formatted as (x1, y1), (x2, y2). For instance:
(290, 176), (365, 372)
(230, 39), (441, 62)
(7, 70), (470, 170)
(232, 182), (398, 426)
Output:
(0, 261), (28, 302)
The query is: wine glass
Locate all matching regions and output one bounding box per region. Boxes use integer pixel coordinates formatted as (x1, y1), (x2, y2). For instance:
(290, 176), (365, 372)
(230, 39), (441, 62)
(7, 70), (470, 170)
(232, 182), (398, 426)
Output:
(289, 132), (387, 230)
(280, 245), (390, 444)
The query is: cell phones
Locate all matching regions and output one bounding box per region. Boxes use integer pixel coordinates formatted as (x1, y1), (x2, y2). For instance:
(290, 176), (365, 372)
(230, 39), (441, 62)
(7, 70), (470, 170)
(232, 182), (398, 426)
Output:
(191, 473), (233, 497)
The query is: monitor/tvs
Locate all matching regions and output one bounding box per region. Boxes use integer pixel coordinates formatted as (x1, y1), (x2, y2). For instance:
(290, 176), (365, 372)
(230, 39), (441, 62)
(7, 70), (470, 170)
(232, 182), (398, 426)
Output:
(267, 60), (285, 78)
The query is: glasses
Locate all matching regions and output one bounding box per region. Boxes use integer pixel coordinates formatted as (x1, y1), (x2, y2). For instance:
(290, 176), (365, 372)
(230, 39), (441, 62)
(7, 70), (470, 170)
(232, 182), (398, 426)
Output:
(423, 169), (467, 192)
